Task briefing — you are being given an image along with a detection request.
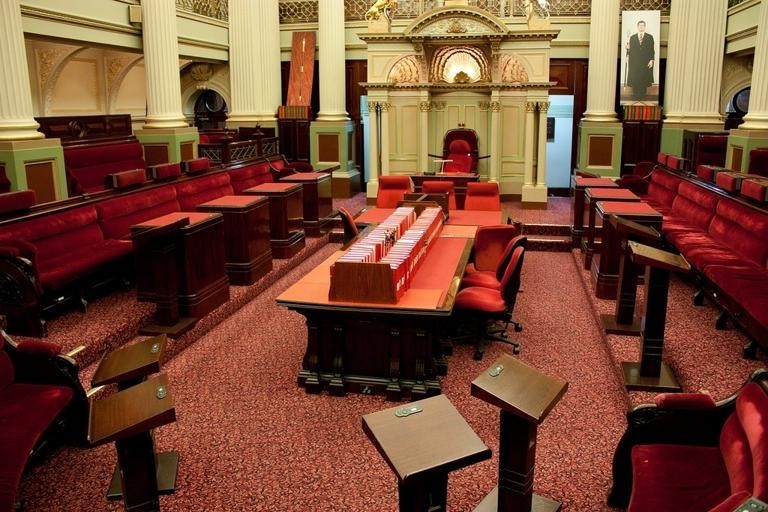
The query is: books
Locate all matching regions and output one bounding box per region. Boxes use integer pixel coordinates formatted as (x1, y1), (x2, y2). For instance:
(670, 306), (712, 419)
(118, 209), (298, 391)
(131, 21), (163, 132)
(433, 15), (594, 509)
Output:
(278, 105), (311, 120)
(623, 103), (660, 121)
(336, 205), (444, 302)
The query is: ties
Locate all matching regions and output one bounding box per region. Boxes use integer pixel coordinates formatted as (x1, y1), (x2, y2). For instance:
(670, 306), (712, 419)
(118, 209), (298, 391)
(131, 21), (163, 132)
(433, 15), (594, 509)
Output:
(639, 33), (643, 46)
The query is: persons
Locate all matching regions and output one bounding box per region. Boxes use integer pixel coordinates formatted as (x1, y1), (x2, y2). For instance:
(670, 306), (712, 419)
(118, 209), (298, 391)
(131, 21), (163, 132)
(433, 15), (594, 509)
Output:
(626, 21), (655, 99)
(547, 118), (553, 136)
